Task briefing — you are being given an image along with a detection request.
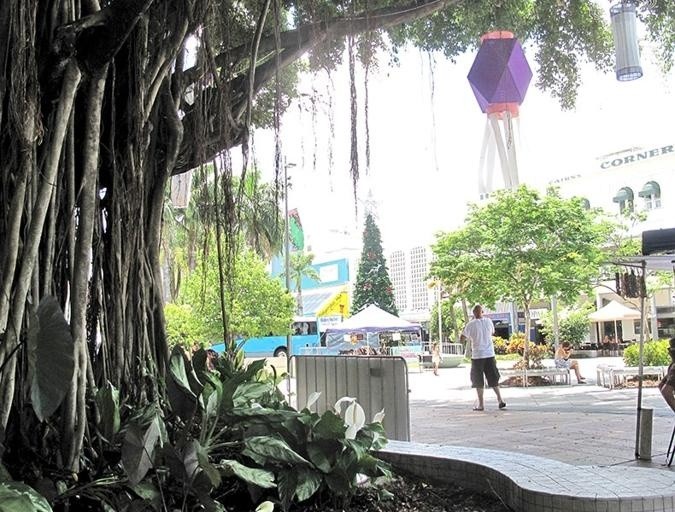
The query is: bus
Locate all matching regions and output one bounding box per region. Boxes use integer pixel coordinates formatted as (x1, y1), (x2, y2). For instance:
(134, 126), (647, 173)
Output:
(209, 315), (322, 358)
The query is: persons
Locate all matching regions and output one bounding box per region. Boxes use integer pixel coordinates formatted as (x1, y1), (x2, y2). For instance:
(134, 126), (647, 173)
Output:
(656, 336), (675, 413)
(602, 334), (613, 350)
(338, 346), (382, 357)
(459, 305), (507, 411)
(430, 340), (443, 376)
(611, 336), (622, 343)
(554, 339), (587, 385)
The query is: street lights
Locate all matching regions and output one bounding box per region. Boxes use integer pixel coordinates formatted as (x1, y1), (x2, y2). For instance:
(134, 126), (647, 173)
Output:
(284, 155), (298, 295)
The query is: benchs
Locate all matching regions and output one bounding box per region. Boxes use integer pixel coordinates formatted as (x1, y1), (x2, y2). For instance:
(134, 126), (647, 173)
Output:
(487, 367), (571, 388)
(595, 364), (670, 390)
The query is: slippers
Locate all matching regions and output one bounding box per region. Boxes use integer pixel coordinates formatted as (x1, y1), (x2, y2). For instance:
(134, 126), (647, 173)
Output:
(473, 406), (484, 410)
(499, 402), (506, 408)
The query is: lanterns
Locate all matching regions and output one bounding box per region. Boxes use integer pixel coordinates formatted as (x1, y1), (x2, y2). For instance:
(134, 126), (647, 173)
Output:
(469, 30), (536, 186)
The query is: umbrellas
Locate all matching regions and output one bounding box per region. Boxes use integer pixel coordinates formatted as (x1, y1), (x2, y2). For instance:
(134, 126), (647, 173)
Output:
(534, 304), (583, 325)
(325, 302), (422, 356)
(588, 299), (652, 344)
